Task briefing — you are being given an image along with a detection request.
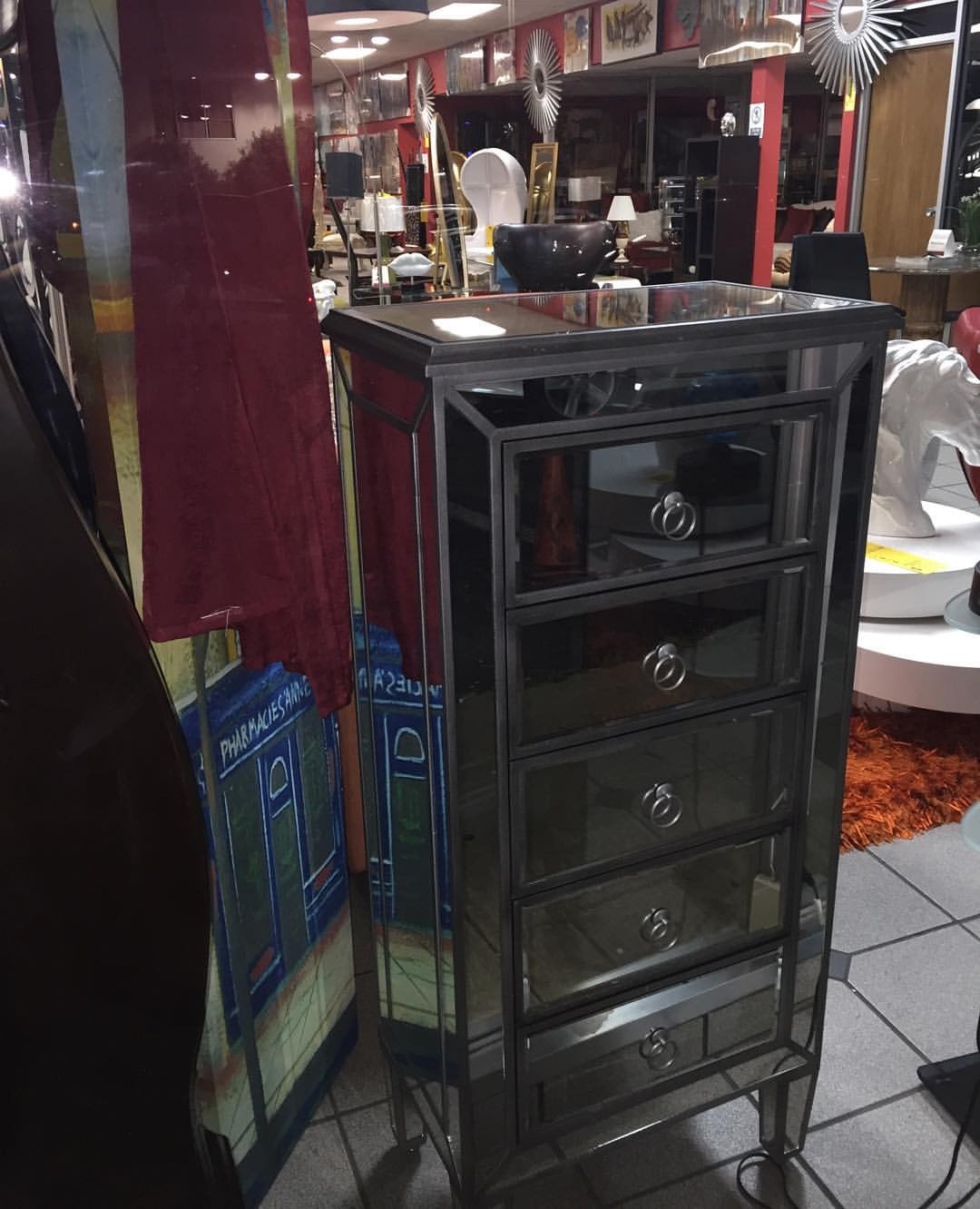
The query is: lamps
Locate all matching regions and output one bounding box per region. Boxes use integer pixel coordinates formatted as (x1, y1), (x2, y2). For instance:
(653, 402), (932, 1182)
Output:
(568, 175), (601, 221)
(360, 199), (406, 288)
(325, 152), (365, 306)
(306, 0), (430, 32)
(607, 196), (638, 263)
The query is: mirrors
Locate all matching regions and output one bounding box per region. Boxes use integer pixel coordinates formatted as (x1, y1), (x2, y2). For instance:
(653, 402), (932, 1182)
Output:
(526, 142), (559, 225)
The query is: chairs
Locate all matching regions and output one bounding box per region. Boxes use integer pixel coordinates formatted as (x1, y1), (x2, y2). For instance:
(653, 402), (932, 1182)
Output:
(772, 202), (905, 340)
(316, 149), (672, 306)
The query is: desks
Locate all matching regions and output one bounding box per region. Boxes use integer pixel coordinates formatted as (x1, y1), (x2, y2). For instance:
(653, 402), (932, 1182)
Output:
(867, 257), (980, 341)
(324, 245), (431, 265)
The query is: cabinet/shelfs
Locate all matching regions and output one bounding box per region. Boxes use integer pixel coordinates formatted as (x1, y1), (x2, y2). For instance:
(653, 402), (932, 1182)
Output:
(680, 133), (758, 286)
(320, 279), (911, 1208)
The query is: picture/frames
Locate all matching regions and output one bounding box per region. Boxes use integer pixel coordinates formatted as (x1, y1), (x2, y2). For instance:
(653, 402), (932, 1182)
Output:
(601, 0), (660, 65)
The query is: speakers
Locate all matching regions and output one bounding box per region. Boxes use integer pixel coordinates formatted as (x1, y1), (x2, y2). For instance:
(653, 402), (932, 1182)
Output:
(405, 164), (424, 206)
(326, 152), (364, 199)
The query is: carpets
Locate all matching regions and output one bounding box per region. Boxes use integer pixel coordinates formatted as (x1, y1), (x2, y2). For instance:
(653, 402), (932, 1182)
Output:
(839, 708), (979, 859)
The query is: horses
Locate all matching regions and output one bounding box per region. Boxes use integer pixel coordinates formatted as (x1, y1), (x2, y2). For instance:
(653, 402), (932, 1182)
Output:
(868, 338), (980, 537)
